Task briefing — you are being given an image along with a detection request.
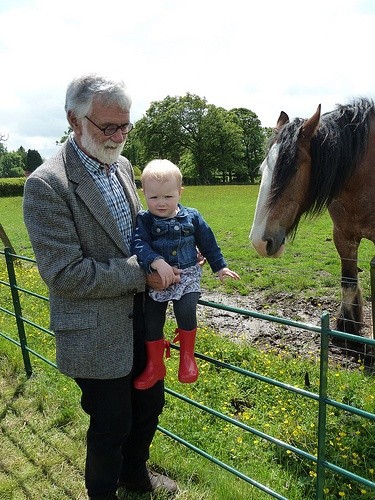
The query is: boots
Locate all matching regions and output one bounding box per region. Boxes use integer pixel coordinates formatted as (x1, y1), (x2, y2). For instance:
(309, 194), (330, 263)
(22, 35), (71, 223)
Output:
(173, 328), (198, 383)
(134, 337), (170, 390)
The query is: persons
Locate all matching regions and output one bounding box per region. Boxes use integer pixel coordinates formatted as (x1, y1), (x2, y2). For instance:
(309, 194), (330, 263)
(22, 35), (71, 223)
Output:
(132, 159), (241, 390)
(22, 73), (207, 500)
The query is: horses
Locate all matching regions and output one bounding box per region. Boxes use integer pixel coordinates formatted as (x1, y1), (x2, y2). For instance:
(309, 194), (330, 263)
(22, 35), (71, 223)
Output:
(249, 95), (375, 348)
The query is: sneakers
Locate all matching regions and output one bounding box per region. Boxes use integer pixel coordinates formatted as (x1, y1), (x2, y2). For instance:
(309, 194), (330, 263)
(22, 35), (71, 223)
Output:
(121, 468), (178, 495)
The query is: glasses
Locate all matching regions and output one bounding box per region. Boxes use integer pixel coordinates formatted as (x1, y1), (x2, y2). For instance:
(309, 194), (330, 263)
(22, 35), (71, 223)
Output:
(85, 115), (133, 136)
